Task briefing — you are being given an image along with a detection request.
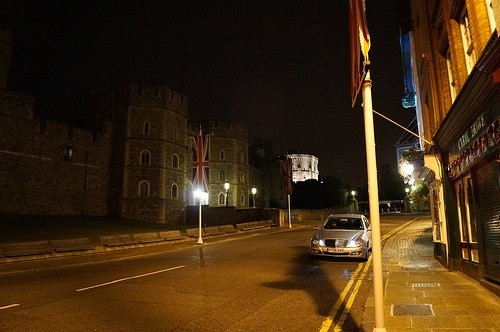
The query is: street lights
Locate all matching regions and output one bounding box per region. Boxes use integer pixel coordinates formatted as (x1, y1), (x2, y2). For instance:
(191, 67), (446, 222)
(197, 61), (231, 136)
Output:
(251, 185), (257, 208)
(224, 180), (230, 206)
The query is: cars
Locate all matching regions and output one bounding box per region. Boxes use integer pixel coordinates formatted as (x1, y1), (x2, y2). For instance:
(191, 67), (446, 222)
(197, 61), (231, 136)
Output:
(311, 213), (372, 261)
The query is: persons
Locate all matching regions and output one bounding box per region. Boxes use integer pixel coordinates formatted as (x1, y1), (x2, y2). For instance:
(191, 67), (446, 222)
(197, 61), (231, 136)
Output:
(354, 220), (362, 230)
